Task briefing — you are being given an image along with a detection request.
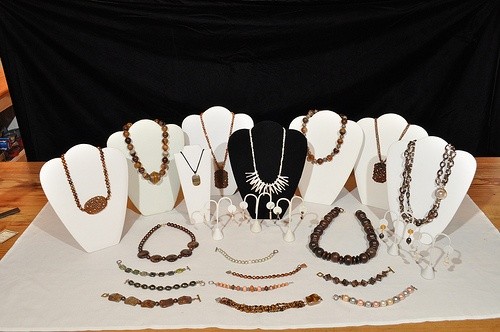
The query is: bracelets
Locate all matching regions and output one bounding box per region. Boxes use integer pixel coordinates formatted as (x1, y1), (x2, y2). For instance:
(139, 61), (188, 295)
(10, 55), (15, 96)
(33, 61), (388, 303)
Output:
(101, 247), (418, 313)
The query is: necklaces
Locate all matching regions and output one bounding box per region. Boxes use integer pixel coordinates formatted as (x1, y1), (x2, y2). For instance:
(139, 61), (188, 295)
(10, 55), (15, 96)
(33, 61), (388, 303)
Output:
(60, 111), (457, 265)
